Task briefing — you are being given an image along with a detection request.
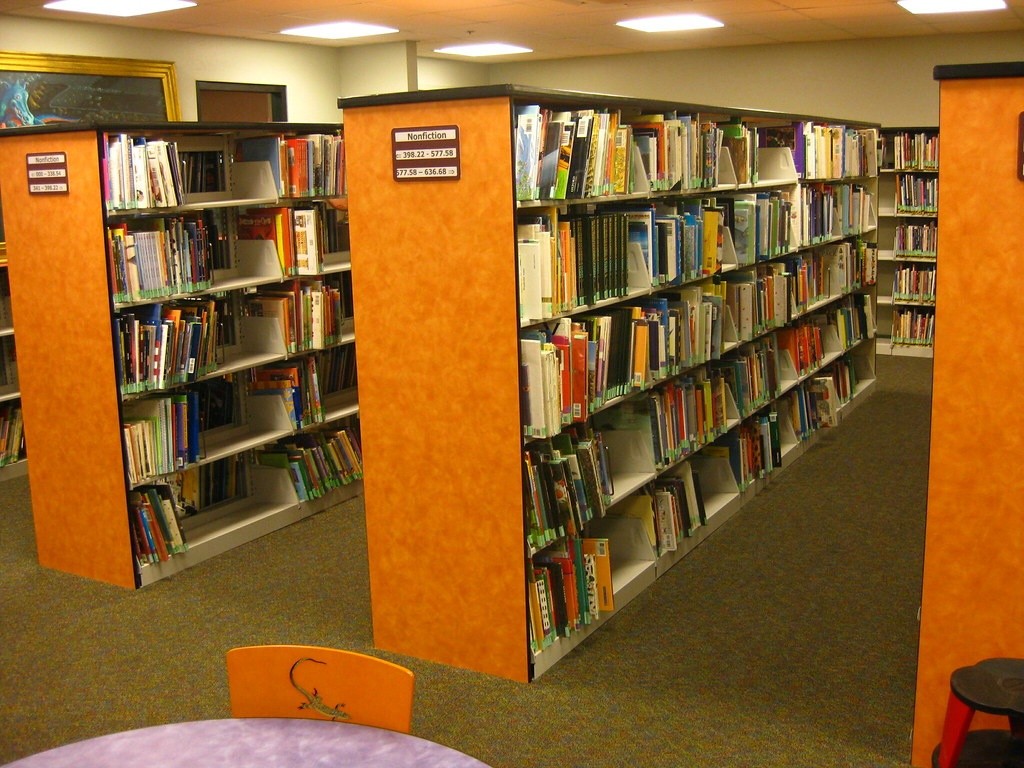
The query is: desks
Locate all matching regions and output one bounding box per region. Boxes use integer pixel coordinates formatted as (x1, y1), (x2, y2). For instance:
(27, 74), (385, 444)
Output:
(0, 717), (504, 768)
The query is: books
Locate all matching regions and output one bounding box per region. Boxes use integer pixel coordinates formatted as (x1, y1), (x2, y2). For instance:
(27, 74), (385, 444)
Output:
(0, 104), (887, 658)
(891, 132), (940, 347)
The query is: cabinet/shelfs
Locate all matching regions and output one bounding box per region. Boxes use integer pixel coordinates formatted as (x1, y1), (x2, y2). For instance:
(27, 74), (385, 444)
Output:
(0, 60), (1024, 768)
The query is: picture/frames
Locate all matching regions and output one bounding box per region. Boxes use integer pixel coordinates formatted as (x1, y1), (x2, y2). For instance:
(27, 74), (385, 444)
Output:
(0, 55), (181, 133)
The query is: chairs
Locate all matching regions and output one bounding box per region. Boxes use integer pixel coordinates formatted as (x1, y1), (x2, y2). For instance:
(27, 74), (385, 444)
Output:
(224, 643), (415, 732)
